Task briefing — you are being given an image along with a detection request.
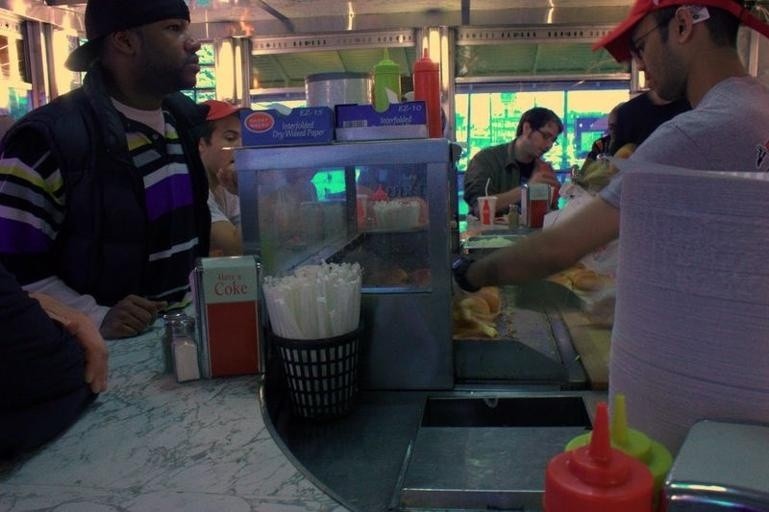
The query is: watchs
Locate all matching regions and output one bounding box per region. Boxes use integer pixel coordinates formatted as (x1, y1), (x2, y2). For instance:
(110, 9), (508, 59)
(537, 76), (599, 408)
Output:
(451, 255), (481, 293)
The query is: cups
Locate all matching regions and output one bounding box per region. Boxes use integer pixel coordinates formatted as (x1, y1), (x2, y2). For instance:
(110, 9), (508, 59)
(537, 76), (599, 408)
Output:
(477, 196), (498, 227)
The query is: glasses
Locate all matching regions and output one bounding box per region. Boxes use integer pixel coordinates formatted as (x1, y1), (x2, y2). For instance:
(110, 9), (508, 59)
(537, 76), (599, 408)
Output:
(536, 128), (559, 146)
(630, 14), (673, 59)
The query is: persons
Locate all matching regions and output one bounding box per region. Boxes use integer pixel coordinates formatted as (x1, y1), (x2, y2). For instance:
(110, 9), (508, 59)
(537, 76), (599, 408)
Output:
(0, 259), (110, 464)
(576, 101), (625, 175)
(610, 89), (693, 158)
(451, 0), (769, 294)
(462, 104), (564, 219)
(198, 97), (254, 256)
(0, 0), (214, 343)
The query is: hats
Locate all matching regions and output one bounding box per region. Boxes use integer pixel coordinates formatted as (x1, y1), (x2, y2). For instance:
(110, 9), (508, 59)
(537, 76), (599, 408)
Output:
(64, 0), (190, 72)
(593, 0), (769, 63)
(201, 101), (252, 120)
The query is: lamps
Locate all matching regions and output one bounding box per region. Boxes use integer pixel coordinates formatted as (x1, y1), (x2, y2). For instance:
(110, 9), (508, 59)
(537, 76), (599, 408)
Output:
(422, 24), (450, 95)
(214, 36), (244, 104)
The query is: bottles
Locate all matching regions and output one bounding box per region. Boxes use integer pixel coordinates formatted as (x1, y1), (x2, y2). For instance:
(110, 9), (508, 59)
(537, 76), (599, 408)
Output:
(542, 393), (674, 512)
(507, 204), (520, 228)
(374, 47), (442, 138)
(162, 310), (201, 382)
